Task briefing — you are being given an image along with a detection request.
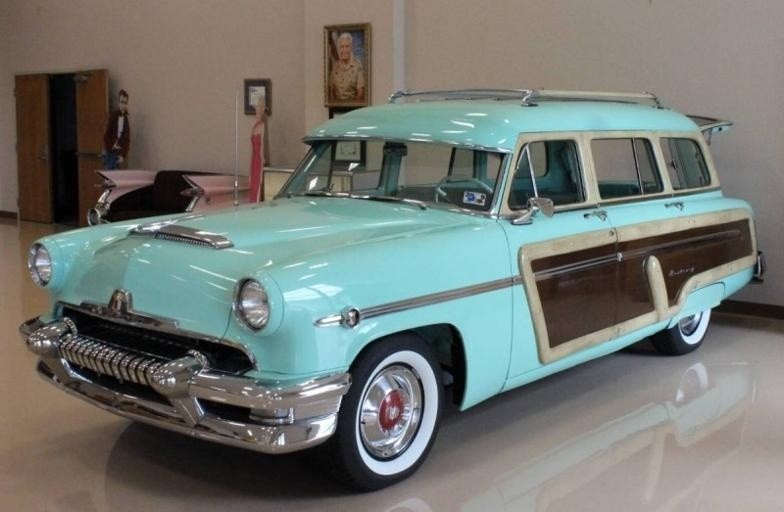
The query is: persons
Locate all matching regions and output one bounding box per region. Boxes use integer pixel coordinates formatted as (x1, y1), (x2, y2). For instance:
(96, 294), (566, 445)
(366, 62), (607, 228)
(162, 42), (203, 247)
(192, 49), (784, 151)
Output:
(328, 32), (365, 100)
(101, 89), (132, 170)
(247, 94), (266, 202)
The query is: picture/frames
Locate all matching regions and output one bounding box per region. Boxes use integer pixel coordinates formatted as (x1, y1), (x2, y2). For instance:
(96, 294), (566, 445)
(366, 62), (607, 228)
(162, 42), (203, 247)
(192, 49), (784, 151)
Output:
(323, 23), (373, 108)
(244, 78), (272, 114)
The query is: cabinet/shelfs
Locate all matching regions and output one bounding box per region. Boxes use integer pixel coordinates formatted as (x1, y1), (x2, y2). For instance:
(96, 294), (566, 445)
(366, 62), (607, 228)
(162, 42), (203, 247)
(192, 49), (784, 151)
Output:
(264, 168), (379, 200)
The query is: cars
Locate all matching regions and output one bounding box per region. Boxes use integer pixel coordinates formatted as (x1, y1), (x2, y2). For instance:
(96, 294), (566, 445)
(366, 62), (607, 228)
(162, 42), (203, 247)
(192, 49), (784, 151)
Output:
(16, 86), (768, 494)
